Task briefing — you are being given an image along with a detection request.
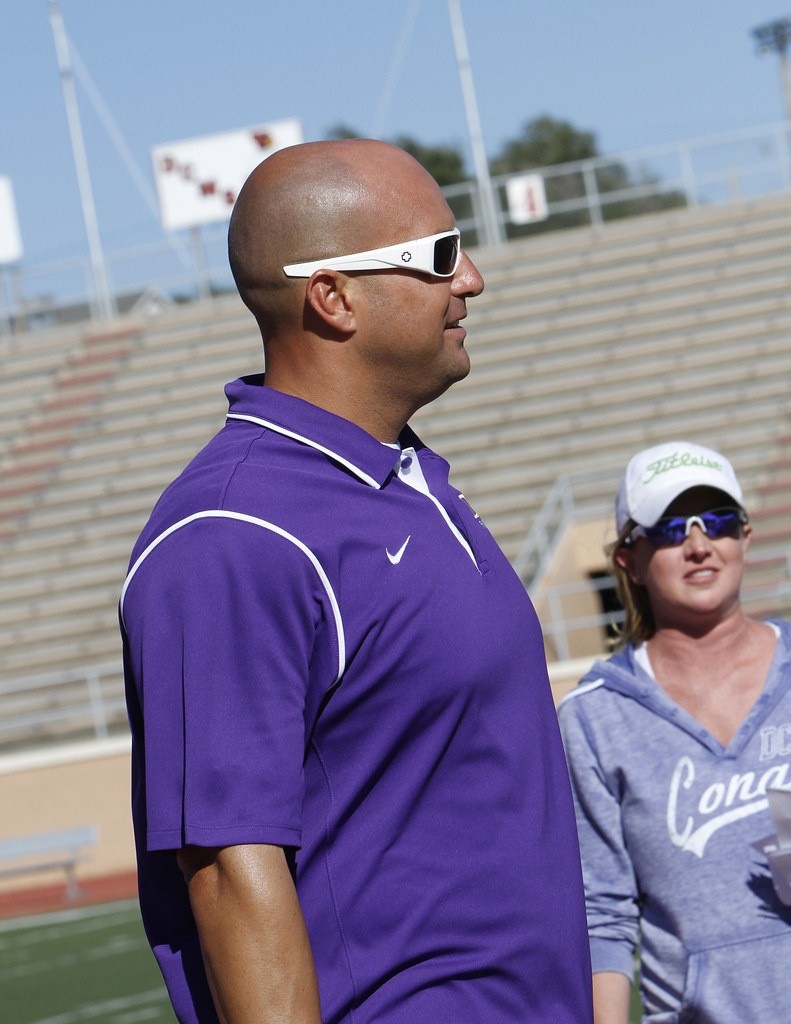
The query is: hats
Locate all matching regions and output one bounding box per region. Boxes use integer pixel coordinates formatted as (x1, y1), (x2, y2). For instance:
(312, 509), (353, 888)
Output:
(617, 440), (747, 541)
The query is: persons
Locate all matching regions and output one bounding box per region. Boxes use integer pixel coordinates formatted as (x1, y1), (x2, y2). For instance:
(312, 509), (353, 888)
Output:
(557, 442), (791, 1024)
(117, 141), (596, 1024)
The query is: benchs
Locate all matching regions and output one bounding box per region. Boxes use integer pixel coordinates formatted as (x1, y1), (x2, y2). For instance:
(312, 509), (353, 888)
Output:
(0, 820), (96, 900)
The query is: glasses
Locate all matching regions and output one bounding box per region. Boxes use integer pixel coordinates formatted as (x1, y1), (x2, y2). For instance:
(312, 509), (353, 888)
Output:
(618, 505), (749, 550)
(282, 226), (462, 277)
